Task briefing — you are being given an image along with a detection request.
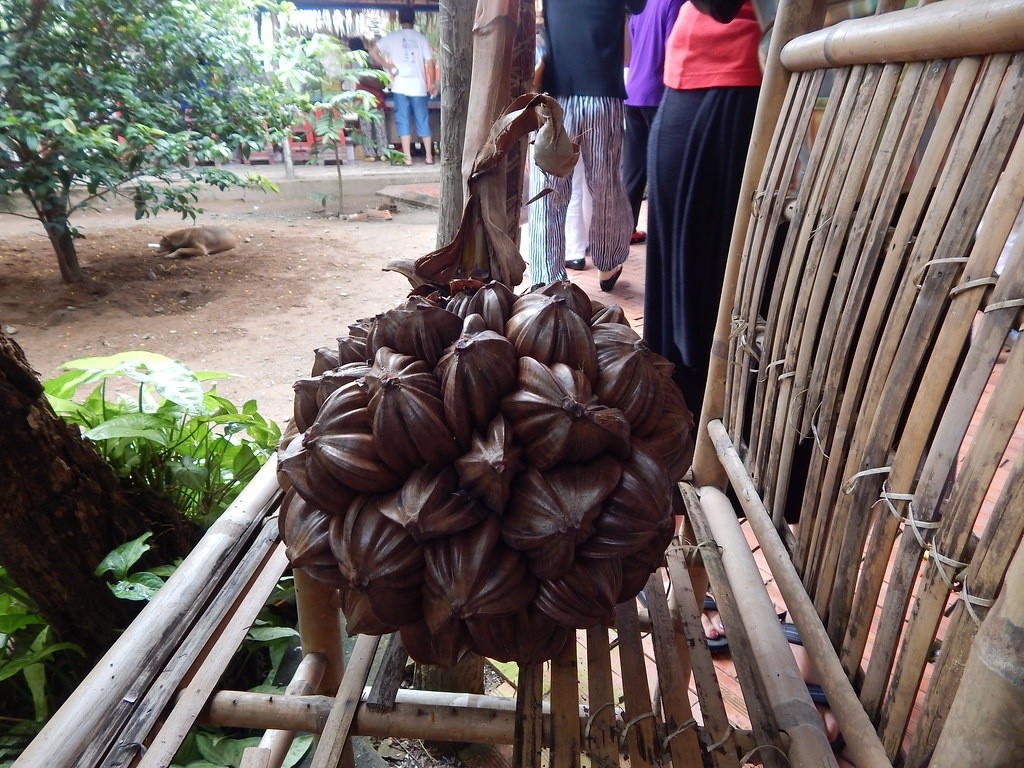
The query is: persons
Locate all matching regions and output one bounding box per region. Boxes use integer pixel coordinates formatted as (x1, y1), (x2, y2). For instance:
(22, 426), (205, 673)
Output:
(529, 0), (780, 424)
(346, 8), (435, 166)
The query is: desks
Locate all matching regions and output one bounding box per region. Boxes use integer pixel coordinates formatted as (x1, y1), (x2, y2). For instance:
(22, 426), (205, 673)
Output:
(353, 93), (441, 160)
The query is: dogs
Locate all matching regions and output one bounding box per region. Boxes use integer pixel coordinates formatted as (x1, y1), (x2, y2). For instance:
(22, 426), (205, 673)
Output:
(156, 226), (237, 259)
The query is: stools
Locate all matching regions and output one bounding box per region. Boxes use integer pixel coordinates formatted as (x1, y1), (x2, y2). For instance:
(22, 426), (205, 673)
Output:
(184, 108), (347, 167)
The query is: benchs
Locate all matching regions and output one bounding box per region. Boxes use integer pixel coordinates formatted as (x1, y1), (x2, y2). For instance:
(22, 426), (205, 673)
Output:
(13, 0), (1024, 768)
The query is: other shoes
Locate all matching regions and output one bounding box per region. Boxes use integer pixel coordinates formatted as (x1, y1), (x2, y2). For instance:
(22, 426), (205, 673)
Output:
(391, 159), (411, 166)
(599, 264), (623, 292)
(425, 157), (435, 165)
(380, 155), (389, 161)
(364, 156), (375, 162)
(630, 231), (646, 244)
(564, 257), (586, 270)
